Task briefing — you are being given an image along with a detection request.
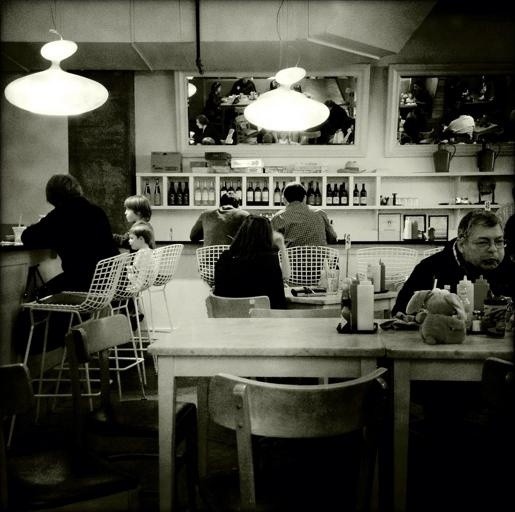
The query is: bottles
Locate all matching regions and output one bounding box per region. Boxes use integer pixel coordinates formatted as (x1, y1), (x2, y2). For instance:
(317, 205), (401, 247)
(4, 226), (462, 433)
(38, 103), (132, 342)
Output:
(360, 184), (367, 204)
(326, 184), (332, 206)
(273, 181), (281, 206)
(254, 182), (262, 206)
(413, 219), (418, 239)
(228, 180), (235, 201)
(315, 182), (322, 206)
(472, 310), (482, 334)
(373, 262), (380, 292)
(356, 274), (374, 330)
(475, 275), (491, 309)
(236, 180), (242, 207)
(353, 184), (360, 205)
(176, 182), (183, 205)
(343, 277), (350, 288)
(456, 275), (473, 332)
(282, 181), (287, 206)
(183, 182), (189, 205)
(404, 216), (412, 239)
(262, 181), (269, 206)
(154, 179), (161, 206)
(341, 182), (348, 206)
(220, 180), (228, 200)
(379, 258), (386, 292)
(144, 179), (151, 205)
(444, 284), (451, 292)
(168, 182), (176, 205)
(307, 181), (315, 206)
(202, 180), (208, 206)
(343, 285), (348, 298)
(333, 183), (340, 206)
(208, 180), (215, 205)
(194, 180), (201, 206)
(247, 181), (254, 205)
(341, 297), (352, 332)
(351, 273), (359, 323)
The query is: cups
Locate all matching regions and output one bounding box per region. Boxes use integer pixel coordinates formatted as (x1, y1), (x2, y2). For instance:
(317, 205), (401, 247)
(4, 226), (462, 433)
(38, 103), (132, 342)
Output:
(326, 269), (338, 295)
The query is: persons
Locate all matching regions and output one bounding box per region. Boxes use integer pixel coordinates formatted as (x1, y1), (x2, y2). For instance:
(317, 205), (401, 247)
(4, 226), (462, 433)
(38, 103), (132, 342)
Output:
(270, 182), (338, 285)
(109, 226), (159, 330)
(123, 195), (158, 252)
(205, 82), (228, 114)
(403, 81), (434, 119)
(226, 78), (257, 98)
(189, 191), (250, 284)
(436, 109), (476, 145)
(213, 214), (288, 309)
(308, 100), (355, 144)
(188, 115), (221, 146)
(392, 208), (515, 337)
(21, 174), (126, 302)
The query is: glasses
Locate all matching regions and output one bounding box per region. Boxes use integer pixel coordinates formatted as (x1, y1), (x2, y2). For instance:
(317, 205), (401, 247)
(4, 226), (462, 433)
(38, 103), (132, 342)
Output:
(475, 238), (509, 249)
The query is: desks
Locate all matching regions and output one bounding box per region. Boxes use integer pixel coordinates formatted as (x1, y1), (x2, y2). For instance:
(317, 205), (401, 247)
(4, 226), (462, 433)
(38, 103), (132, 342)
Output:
(1, 241), (69, 390)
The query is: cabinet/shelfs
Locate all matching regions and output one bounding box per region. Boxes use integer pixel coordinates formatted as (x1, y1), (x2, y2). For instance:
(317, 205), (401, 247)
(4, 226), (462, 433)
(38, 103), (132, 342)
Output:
(132, 240), (447, 357)
(136, 173), (515, 211)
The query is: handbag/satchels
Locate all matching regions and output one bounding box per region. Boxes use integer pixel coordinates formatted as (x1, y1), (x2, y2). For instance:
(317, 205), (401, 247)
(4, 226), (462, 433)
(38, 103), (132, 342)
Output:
(10, 263), (69, 355)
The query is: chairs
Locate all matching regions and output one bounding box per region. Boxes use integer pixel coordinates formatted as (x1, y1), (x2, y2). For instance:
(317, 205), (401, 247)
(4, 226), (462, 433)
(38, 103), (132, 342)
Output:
(113, 250), (142, 301)
(197, 367), (391, 512)
(142, 243), (185, 374)
(277, 245), (343, 305)
(422, 247), (445, 260)
(6, 250), (129, 452)
(197, 244), (232, 289)
(64, 312), (193, 497)
(139, 248), (162, 293)
(353, 248), (416, 319)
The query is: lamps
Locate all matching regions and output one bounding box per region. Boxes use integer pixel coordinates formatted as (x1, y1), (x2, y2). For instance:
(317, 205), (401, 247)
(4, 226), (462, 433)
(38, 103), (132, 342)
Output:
(4, 30), (108, 117)
(245, 67), (330, 131)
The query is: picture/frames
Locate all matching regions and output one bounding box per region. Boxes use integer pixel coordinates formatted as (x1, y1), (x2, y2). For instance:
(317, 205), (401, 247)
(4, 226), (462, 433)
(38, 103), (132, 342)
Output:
(384, 62), (515, 159)
(175, 63), (370, 159)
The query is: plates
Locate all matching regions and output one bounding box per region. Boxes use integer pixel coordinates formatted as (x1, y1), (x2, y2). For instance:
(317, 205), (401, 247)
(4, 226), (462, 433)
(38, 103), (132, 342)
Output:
(337, 321), (379, 334)
